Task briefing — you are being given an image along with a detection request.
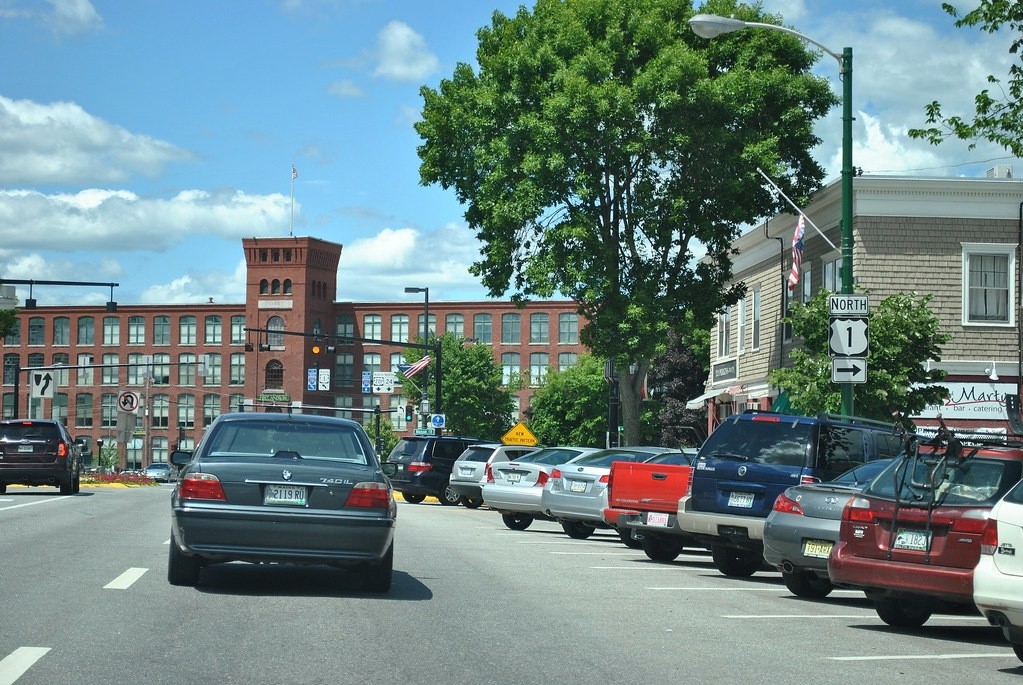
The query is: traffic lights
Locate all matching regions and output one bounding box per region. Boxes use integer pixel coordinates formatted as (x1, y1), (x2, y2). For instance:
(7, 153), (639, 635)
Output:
(328, 347), (337, 353)
(312, 345), (321, 355)
(406, 406), (413, 422)
(262, 344), (270, 350)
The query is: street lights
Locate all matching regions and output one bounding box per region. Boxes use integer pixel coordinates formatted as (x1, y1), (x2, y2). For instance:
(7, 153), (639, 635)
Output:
(688, 11), (858, 416)
(404, 287), (429, 433)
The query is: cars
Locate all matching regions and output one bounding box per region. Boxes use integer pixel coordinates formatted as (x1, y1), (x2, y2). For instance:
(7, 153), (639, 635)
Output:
(0, 418), (85, 495)
(142, 463), (177, 483)
(166, 411), (398, 596)
(385, 409), (1023, 663)
